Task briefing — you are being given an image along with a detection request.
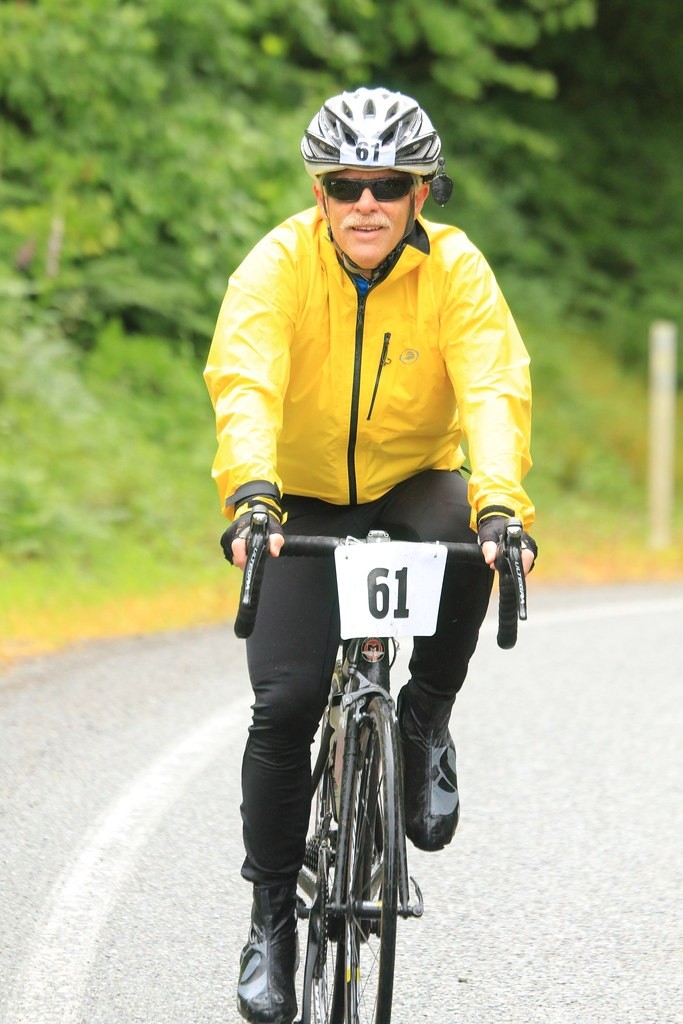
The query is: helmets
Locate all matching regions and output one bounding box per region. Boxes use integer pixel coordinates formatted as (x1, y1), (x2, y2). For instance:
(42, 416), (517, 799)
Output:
(300, 86), (441, 176)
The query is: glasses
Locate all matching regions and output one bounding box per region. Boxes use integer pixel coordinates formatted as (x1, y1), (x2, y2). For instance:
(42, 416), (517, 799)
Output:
(321, 174), (437, 202)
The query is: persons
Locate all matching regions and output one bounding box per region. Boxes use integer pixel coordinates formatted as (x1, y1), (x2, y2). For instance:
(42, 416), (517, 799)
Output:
(203, 87), (538, 1024)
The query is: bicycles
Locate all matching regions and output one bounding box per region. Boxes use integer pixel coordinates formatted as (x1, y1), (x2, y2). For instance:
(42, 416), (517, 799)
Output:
(233, 502), (531, 1024)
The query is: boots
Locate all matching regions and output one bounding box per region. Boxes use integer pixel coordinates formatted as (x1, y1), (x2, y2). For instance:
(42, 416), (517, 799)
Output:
(394, 683), (459, 852)
(235, 875), (301, 1024)
